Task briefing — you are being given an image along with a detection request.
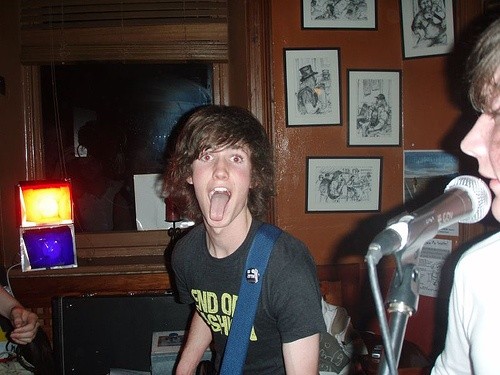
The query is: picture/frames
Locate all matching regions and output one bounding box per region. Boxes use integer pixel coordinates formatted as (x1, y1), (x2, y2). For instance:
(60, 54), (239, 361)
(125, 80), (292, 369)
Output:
(402, 149), (460, 212)
(300, 0), (378, 31)
(305, 156), (383, 213)
(282, 47), (343, 128)
(346, 68), (403, 148)
(400, 0), (457, 60)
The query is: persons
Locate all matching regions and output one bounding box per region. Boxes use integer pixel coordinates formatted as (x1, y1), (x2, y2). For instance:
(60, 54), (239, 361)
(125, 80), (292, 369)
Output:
(432, 20), (500, 375)
(160, 105), (326, 375)
(0, 286), (39, 375)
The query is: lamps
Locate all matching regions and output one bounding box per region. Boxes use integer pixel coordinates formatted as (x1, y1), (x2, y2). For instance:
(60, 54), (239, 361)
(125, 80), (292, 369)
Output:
(16, 179), (78, 273)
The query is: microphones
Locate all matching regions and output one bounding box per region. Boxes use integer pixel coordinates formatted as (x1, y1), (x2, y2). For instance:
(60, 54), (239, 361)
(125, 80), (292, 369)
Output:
(367, 175), (493, 264)
